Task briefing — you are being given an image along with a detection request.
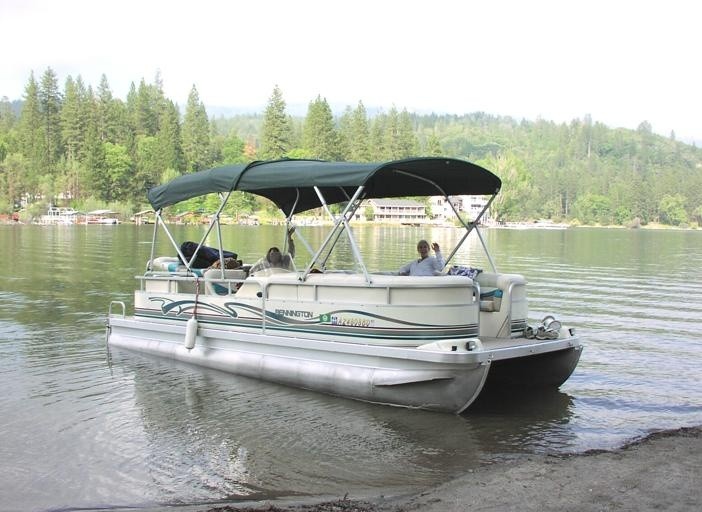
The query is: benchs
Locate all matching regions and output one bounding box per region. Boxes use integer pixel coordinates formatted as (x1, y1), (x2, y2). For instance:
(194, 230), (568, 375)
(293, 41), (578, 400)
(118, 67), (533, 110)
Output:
(476, 270), (527, 337)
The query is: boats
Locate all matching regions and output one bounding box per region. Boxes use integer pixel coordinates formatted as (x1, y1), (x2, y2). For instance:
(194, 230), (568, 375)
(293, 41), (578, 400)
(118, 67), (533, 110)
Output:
(105, 157), (584, 418)
(30, 205), (120, 226)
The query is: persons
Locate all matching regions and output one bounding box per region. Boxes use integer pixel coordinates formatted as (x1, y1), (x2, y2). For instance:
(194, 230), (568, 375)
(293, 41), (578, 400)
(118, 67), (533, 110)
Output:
(400, 239), (443, 275)
(248, 226), (296, 273)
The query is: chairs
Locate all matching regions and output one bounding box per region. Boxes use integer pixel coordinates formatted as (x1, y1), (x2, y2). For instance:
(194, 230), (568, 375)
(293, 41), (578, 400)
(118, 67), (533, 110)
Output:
(204, 268), (246, 298)
